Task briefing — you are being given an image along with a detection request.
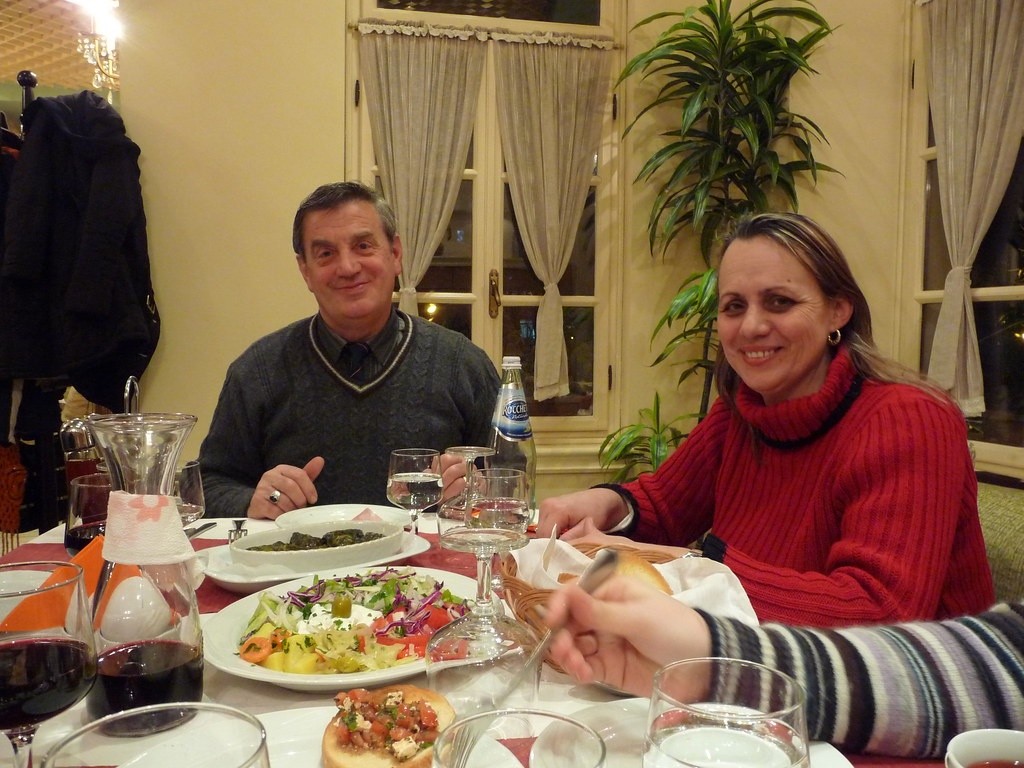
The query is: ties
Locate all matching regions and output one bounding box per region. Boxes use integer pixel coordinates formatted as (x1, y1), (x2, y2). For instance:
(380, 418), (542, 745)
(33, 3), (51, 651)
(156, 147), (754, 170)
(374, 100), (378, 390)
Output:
(343, 340), (369, 388)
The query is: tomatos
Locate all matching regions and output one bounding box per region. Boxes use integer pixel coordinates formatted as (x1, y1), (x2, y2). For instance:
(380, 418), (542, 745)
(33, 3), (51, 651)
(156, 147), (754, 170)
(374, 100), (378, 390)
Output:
(359, 603), (467, 661)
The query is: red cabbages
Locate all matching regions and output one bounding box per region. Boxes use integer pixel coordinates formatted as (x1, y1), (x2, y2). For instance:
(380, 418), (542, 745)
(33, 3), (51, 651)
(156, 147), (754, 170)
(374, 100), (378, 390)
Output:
(284, 567), (474, 634)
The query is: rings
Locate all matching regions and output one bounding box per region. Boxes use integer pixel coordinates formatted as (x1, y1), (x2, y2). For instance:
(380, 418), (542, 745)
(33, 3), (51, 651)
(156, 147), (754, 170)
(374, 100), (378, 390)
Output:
(268, 490), (280, 503)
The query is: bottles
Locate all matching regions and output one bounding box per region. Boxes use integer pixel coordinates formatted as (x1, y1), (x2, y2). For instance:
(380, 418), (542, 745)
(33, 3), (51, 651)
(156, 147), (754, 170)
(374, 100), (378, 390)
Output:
(484, 357), (536, 525)
(81, 412), (205, 736)
(60, 418), (112, 528)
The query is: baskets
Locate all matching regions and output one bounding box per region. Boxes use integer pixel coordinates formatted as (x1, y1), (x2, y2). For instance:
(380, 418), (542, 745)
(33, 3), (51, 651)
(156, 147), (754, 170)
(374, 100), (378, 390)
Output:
(501, 543), (680, 675)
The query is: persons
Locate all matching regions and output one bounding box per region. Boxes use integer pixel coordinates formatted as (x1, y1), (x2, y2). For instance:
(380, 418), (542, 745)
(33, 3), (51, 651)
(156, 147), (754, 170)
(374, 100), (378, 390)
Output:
(179, 182), (504, 521)
(547, 561), (1024, 761)
(537, 212), (998, 627)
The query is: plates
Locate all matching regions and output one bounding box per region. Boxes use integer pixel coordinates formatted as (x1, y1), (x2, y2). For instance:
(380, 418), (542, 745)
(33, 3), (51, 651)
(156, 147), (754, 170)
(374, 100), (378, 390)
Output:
(275, 504), (411, 526)
(196, 531), (431, 593)
(529, 697), (855, 768)
(253, 706), (341, 768)
(203, 566), (505, 691)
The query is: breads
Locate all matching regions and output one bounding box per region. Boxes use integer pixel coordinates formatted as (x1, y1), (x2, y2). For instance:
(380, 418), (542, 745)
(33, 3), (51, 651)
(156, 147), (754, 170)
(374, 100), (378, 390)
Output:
(613, 552), (674, 597)
(321, 683), (456, 768)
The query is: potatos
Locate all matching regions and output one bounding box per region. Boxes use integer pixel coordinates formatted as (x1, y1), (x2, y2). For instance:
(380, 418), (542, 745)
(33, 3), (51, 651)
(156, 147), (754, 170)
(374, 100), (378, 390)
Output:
(250, 622), (324, 674)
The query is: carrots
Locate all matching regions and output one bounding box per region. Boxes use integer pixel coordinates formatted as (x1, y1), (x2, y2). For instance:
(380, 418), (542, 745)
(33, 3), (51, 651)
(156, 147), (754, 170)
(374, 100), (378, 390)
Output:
(271, 626), (290, 650)
(241, 637), (273, 662)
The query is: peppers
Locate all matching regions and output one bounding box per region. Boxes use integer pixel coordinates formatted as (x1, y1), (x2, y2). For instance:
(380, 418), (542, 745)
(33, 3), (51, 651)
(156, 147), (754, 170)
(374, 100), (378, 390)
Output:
(245, 528), (386, 551)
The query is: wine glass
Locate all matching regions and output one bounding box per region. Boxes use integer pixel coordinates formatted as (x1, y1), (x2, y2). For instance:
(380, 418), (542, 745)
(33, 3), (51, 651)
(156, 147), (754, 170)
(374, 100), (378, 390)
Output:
(386, 448), (444, 535)
(0, 561), (98, 768)
(425, 528), (542, 723)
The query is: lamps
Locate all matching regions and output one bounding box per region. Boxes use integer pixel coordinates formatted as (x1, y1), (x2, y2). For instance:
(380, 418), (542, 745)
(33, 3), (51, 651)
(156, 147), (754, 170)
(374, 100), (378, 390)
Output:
(69, 0), (125, 105)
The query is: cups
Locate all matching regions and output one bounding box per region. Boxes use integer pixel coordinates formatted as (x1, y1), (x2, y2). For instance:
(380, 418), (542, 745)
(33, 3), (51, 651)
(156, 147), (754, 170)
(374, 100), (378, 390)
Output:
(40, 702), (271, 768)
(439, 446), (497, 520)
(466, 469), (529, 533)
(432, 709), (607, 768)
(64, 461), (205, 557)
(643, 656), (810, 768)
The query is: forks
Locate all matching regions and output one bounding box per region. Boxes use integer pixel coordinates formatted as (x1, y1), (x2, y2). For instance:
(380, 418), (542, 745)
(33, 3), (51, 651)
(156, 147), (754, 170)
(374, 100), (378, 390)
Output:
(447, 549), (618, 768)
(228, 520), (248, 543)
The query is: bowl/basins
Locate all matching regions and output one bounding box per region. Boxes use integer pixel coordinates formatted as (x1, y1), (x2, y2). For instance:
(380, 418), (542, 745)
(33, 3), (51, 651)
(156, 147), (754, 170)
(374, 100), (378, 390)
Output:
(945, 729), (1024, 768)
(229, 522), (404, 573)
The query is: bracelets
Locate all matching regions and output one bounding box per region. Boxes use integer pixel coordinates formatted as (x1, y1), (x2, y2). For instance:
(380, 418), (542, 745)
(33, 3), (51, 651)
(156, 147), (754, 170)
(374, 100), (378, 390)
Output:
(680, 551), (702, 558)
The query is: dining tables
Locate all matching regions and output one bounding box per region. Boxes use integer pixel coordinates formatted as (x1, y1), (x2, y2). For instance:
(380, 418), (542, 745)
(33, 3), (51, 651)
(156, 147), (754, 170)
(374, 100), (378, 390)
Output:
(1, 504), (947, 768)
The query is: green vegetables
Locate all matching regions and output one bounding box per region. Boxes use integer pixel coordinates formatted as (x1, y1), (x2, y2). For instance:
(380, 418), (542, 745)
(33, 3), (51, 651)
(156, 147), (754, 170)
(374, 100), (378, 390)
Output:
(235, 575), (466, 656)
